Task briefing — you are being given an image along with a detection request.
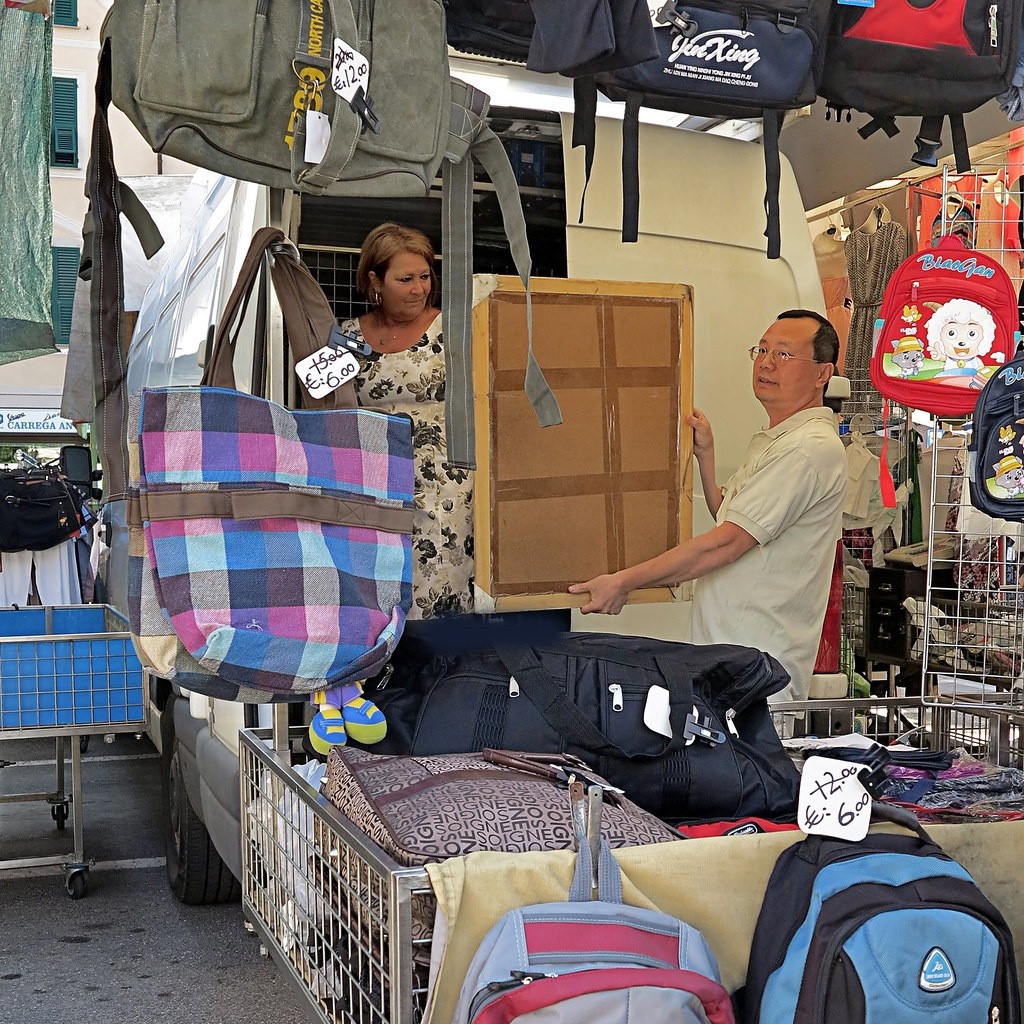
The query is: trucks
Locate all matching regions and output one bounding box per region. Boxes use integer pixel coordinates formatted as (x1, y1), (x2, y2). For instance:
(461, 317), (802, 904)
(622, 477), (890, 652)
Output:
(61, 102), (1024, 1024)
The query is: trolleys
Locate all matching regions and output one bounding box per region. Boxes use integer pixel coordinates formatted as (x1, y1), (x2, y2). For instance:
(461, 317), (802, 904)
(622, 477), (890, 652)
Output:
(0, 603), (148, 899)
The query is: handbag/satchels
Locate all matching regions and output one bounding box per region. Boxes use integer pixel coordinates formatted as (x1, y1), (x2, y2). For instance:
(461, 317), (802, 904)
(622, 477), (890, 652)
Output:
(316, 743), (694, 983)
(346, 627), (802, 825)
(450, 834), (734, 1024)
(124, 226), (416, 756)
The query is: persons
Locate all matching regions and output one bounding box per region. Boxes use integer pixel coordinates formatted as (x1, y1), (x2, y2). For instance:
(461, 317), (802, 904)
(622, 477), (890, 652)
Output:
(568, 310), (848, 739)
(342, 223), (474, 620)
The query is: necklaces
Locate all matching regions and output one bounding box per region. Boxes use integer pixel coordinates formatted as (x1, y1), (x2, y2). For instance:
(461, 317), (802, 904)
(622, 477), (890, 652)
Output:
(377, 314), (415, 345)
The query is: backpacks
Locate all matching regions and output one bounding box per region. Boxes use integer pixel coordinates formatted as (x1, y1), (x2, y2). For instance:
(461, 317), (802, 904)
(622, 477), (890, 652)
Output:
(571, 0), (832, 260)
(799, 743), (1024, 823)
(967, 357), (1024, 523)
(816, 0), (1024, 174)
(869, 233), (1020, 508)
(77, 0), (564, 507)
(735, 801), (1022, 1024)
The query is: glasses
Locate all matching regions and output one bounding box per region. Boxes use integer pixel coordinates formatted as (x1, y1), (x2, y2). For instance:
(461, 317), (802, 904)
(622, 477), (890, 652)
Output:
(748, 346), (826, 367)
